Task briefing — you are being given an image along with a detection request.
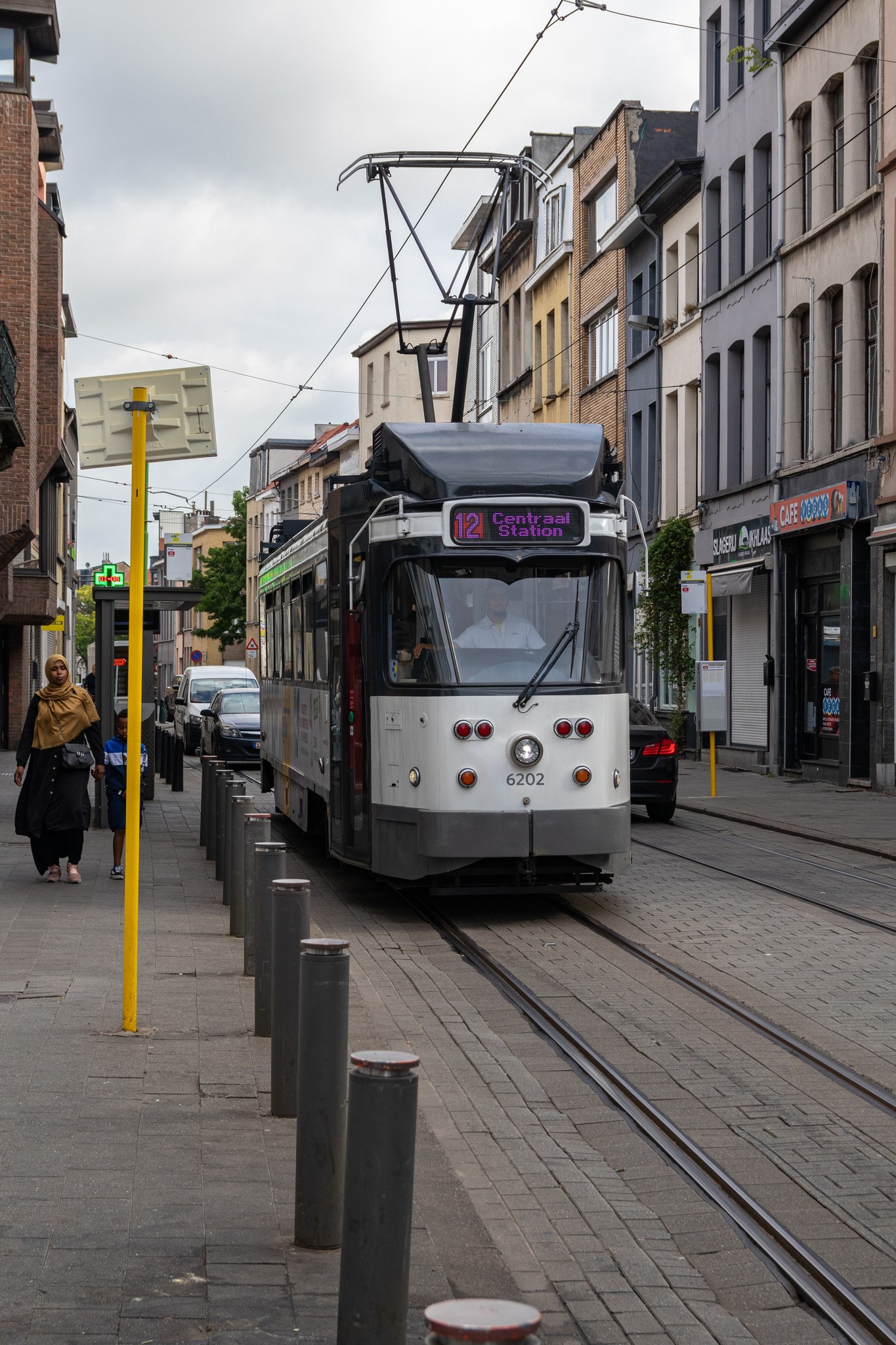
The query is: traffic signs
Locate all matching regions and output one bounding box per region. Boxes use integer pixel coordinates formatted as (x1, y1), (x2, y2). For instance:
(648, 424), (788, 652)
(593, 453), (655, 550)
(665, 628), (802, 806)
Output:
(113, 659), (127, 666)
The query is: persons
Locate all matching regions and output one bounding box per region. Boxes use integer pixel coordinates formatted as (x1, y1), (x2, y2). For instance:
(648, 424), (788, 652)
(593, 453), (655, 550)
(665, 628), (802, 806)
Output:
(413, 581), (547, 660)
(819, 667), (840, 734)
(91, 709), (148, 880)
(83, 664), (96, 705)
(13, 654), (108, 884)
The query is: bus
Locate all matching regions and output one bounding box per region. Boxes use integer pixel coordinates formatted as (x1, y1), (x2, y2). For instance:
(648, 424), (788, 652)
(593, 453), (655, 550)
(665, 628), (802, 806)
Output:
(256, 147), (649, 903)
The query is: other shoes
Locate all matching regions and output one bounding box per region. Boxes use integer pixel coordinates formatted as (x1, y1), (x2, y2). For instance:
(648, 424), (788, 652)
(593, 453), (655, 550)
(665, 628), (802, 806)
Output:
(65, 860), (81, 883)
(45, 865), (62, 882)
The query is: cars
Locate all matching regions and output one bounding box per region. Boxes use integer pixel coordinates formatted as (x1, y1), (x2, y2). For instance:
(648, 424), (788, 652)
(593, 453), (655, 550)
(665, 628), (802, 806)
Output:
(162, 675), (183, 722)
(197, 688), (261, 769)
(629, 699), (682, 823)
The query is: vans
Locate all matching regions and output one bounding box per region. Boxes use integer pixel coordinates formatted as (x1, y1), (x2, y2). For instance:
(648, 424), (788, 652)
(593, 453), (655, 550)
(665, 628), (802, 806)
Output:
(174, 665), (258, 756)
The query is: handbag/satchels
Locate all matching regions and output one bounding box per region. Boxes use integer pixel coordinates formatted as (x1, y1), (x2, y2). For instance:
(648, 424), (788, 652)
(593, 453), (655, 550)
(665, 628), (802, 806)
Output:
(63, 742), (94, 768)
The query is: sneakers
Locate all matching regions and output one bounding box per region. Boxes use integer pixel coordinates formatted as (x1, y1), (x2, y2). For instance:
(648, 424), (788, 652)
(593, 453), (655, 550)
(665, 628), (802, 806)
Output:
(110, 866), (124, 879)
